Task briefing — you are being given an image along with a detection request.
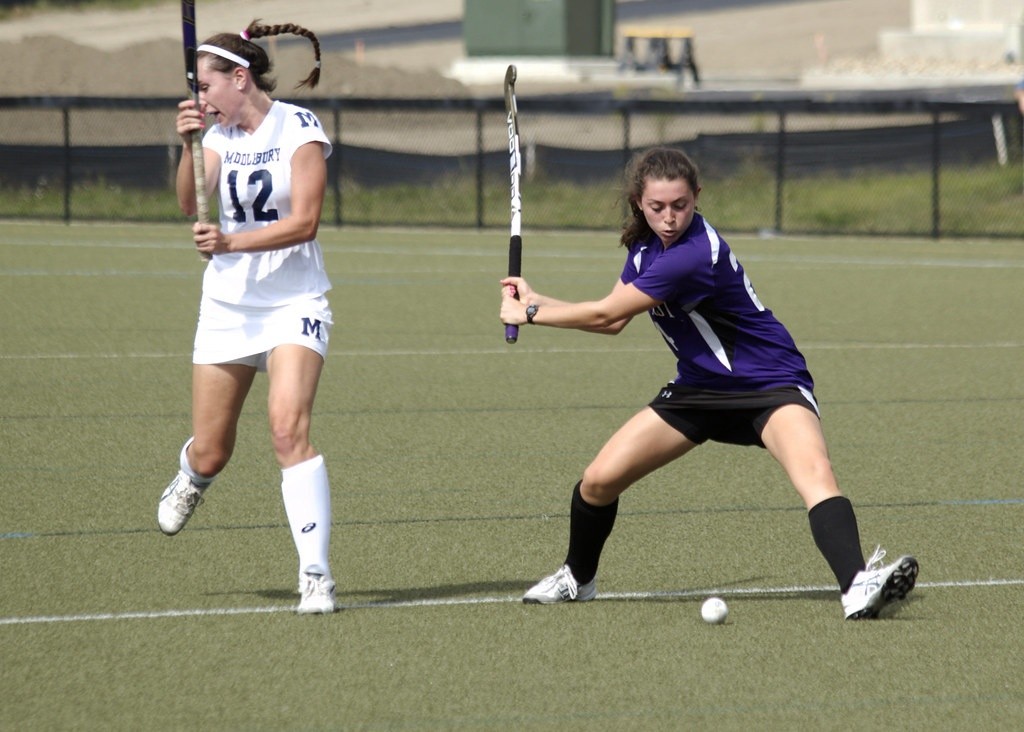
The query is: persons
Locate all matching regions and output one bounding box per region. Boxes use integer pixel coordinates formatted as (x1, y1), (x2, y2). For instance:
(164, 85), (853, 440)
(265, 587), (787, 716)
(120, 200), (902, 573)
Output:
(158, 24), (340, 613)
(501, 150), (919, 618)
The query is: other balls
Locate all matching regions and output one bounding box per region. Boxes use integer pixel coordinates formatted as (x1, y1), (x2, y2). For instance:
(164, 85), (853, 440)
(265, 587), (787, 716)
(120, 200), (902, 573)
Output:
(699, 596), (730, 624)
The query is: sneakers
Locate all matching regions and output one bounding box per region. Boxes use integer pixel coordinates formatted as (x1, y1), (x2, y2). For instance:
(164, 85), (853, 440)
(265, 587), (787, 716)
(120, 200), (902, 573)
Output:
(522, 565), (597, 605)
(299, 564), (337, 615)
(837, 544), (920, 621)
(157, 470), (207, 537)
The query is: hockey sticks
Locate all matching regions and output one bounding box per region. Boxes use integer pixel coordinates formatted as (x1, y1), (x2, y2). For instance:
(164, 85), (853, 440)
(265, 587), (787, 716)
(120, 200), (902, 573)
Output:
(500, 64), (524, 343)
(181, 0), (214, 263)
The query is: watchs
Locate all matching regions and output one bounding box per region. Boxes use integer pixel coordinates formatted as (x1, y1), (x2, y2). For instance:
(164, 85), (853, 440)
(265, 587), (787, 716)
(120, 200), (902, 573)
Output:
(526, 305), (540, 325)
